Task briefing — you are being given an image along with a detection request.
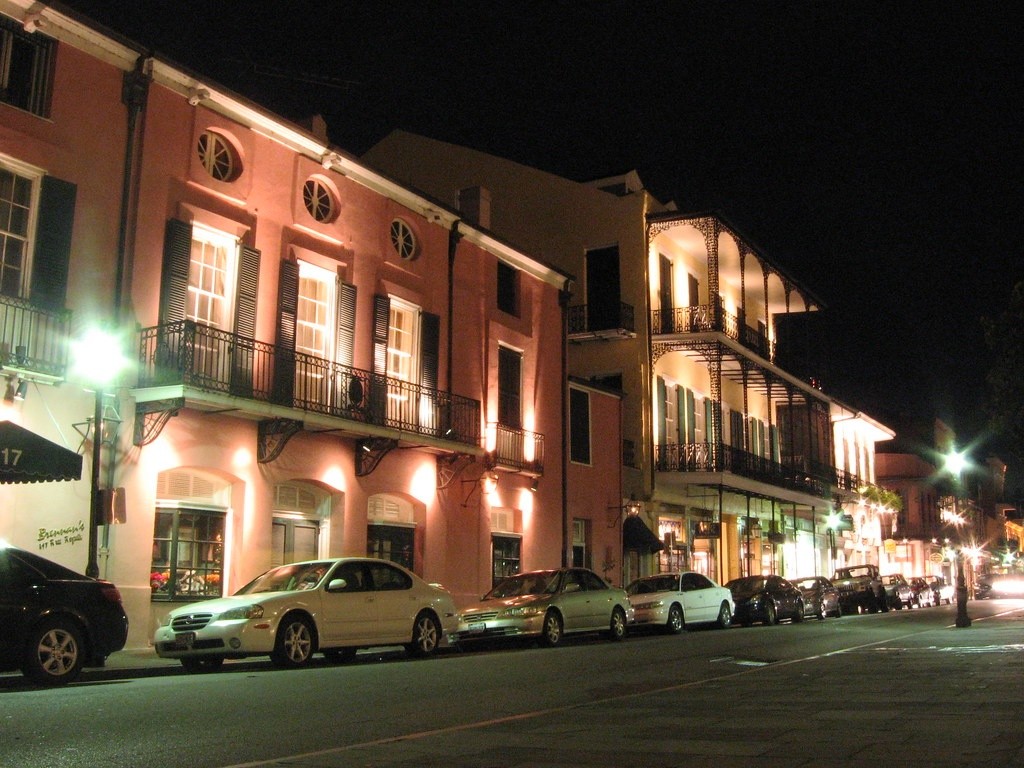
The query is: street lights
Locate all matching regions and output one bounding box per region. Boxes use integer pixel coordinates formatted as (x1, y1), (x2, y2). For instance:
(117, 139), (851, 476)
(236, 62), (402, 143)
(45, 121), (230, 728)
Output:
(75, 326), (122, 579)
(946, 452), (972, 629)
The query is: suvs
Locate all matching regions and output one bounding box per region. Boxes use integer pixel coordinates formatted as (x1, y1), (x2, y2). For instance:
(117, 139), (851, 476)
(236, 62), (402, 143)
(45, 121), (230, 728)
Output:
(828, 565), (888, 614)
(971, 573), (1001, 600)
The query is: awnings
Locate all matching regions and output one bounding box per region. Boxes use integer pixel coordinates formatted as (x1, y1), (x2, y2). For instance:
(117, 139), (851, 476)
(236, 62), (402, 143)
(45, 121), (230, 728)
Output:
(0, 420), (82, 484)
(624, 517), (667, 554)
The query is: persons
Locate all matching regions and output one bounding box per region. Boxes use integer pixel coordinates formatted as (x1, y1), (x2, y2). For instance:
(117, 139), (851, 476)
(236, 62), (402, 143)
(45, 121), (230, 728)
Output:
(298, 565), (341, 588)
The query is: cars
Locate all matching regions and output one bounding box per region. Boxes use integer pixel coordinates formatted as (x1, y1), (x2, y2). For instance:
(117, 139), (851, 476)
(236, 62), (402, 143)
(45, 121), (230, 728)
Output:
(922, 576), (955, 606)
(0, 548), (129, 685)
(880, 574), (915, 610)
(445, 567), (630, 648)
(624, 572), (735, 635)
(791, 576), (843, 619)
(905, 577), (934, 607)
(724, 575), (804, 627)
(153, 558), (459, 669)
